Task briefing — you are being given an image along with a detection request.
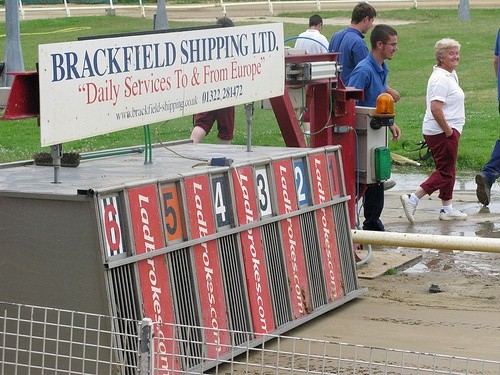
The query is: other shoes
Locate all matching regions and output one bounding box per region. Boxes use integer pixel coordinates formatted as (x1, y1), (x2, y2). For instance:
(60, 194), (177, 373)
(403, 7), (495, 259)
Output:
(384, 180), (396, 190)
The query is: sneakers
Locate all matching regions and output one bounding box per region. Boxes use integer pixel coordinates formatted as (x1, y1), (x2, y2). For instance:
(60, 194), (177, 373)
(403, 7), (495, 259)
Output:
(439, 209), (467, 220)
(475, 173), (491, 205)
(400, 194), (416, 224)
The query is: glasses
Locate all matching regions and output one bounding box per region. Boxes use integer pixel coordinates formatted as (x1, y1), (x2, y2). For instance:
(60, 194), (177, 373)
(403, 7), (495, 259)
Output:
(382, 42), (397, 48)
(320, 21), (323, 29)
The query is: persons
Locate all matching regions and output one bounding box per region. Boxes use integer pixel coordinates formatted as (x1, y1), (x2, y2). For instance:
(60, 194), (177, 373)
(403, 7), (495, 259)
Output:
(346, 24), (401, 231)
(476, 28), (500, 205)
(294, 15), (330, 135)
(400, 38), (467, 224)
(190, 17), (236, 145)
(328, 2), (400, 190)
